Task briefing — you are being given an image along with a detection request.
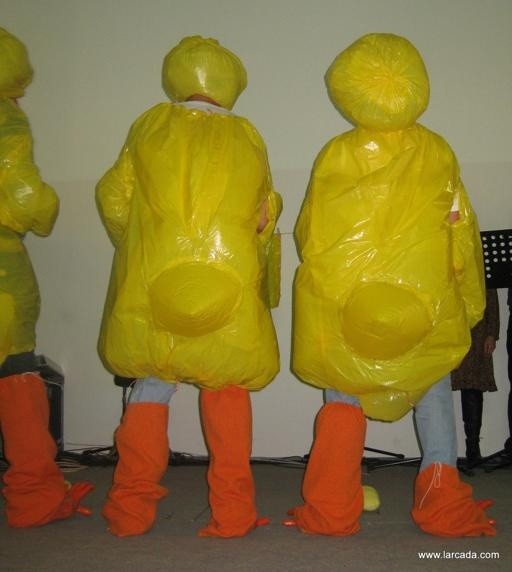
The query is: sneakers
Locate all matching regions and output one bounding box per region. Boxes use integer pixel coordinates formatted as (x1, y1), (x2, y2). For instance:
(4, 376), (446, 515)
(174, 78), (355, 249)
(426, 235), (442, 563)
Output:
(463, 438), (485, 462)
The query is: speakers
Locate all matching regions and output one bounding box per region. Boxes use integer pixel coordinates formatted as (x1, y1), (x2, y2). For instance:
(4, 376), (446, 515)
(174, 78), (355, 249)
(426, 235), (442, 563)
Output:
(36, 354), (65, 458)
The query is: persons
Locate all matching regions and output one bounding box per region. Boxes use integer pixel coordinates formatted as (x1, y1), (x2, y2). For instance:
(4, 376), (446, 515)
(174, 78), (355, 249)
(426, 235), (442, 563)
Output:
(451, 289), (499, 465)
(293, 32), (497, 539)
